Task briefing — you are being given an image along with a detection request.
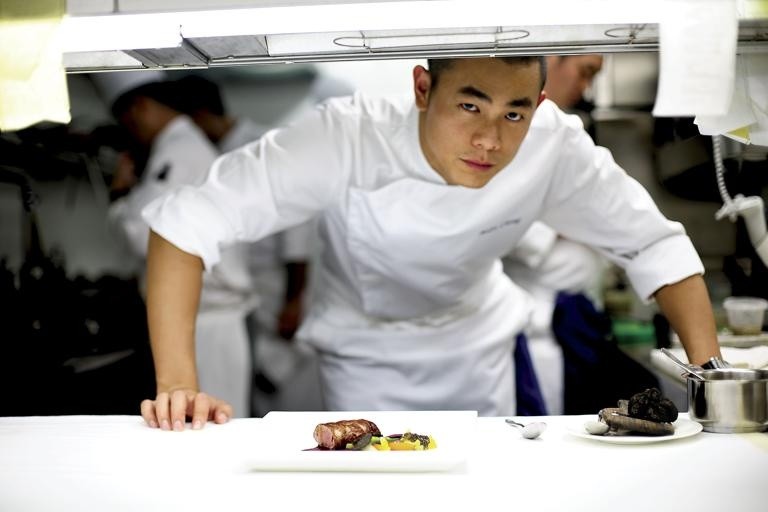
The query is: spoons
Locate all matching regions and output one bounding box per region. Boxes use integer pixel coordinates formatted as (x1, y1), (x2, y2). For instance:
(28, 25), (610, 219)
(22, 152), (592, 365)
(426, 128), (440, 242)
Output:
(505, 419), (547, 440)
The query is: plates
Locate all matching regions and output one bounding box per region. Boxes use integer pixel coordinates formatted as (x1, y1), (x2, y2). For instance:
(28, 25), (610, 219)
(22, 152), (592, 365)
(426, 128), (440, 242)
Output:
(566, 415), (703, 445)
(235, 410), (478, 473)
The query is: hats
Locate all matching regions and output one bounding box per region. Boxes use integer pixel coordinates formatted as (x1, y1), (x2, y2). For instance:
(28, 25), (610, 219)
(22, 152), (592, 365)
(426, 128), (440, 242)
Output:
(89, 71), (169, 114)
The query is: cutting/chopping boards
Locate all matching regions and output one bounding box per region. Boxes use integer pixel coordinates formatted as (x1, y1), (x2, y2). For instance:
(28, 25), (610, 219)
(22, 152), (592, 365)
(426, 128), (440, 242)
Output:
(652, 346), (768, 386)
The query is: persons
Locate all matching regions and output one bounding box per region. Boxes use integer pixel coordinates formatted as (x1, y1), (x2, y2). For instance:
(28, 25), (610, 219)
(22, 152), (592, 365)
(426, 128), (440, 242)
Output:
(495, 56), (618, 415)
(96, 80), (261, 420)
(139, 54), (726, 431)
(63, 117), (153, 202)
(172, 75), (325, 422)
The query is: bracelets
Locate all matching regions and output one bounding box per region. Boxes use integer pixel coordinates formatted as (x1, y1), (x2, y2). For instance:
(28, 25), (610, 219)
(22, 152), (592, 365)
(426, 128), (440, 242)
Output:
(689, 356), (724, 378)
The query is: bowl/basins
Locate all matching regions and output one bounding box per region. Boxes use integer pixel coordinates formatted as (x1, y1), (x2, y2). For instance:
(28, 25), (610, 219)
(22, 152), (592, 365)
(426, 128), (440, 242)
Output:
(681, 367), (768, 432)
